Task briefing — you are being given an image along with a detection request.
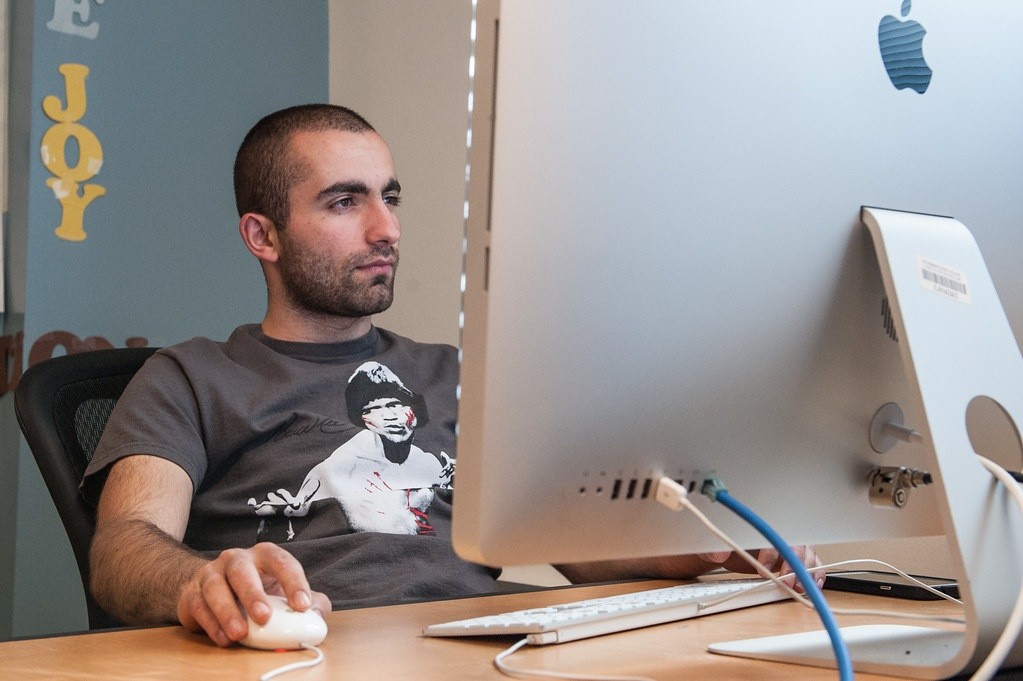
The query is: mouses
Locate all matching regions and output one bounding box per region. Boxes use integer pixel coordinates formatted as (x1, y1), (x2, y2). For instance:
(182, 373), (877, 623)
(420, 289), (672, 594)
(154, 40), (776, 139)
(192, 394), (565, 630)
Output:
(237, 593), (328, 651)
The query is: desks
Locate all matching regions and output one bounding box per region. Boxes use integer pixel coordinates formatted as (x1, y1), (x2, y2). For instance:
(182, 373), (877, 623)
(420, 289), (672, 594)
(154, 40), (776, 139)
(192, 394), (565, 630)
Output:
(0, 577), (971, 681)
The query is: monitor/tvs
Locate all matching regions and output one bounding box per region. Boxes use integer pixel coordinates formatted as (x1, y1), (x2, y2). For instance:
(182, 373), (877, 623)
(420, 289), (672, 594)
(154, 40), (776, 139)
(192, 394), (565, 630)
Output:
(453, 1), (1023, 681)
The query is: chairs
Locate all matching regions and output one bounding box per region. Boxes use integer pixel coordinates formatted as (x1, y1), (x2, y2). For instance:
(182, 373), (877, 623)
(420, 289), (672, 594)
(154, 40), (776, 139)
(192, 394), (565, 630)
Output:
(14, 346), (165, 637)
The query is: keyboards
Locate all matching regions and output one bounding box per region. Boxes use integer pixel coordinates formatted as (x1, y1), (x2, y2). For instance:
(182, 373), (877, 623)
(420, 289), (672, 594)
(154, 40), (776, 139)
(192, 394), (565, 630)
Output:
(423, 563), (821, 644)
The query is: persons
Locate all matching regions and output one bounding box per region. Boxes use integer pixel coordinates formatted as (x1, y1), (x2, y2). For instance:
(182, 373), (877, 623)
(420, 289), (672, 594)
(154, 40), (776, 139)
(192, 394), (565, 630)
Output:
(80, 102), (826, 647)
